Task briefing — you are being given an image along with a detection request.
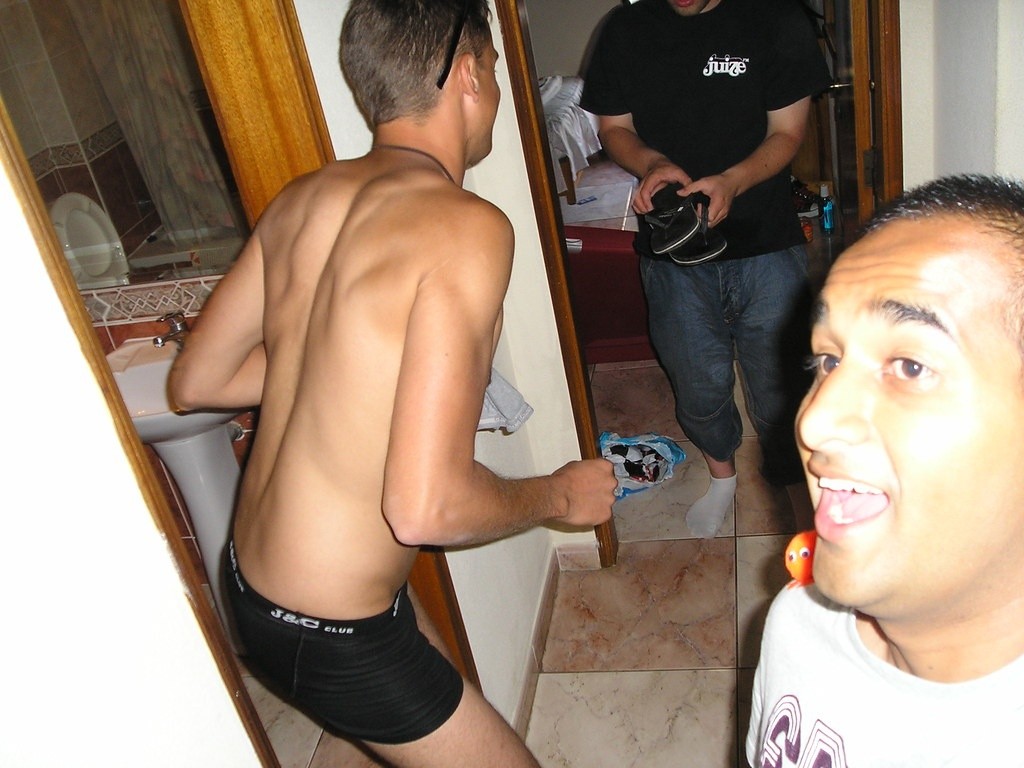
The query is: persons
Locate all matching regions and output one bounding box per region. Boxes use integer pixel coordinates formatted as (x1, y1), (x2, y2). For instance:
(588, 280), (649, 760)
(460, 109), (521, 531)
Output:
(745, 174), (1024, 768)
(167, 1), (619, 768)
(583, 1), (833, 541)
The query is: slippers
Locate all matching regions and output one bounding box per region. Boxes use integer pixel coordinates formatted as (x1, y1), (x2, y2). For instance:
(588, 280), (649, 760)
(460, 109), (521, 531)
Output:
(666, 205), (728, 267)
(645, 191), (700, 256)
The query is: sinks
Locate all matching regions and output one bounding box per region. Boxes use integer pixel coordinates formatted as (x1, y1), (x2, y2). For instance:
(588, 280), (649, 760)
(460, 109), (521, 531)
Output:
(162, 227), (222, 242)
(110, 357), (241, 445)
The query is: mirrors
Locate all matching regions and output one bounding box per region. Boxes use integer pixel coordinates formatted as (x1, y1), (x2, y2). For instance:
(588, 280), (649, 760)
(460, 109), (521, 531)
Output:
(0, 0), (254, 329)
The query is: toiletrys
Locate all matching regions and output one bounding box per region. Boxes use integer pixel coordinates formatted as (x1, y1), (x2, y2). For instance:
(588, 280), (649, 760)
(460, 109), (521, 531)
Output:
(819, 184), (835, 236)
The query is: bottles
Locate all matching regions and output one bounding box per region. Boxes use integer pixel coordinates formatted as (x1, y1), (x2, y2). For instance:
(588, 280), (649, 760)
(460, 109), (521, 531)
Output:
(818, 184), (835, 235)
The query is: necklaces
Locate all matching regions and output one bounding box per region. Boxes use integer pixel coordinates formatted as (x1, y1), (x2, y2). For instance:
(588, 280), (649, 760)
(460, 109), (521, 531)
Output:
(376, 146), (456, 182)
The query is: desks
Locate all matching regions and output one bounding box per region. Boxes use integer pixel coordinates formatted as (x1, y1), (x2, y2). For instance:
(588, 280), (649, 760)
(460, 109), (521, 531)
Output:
(539, 76), (603, 207)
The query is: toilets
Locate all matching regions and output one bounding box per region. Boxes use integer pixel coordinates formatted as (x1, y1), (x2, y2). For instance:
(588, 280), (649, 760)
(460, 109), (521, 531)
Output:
(48, 191), (132, 292)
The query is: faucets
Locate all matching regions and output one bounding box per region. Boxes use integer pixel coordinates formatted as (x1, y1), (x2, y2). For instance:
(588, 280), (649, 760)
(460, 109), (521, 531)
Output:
(152, 309), (190, 348)
